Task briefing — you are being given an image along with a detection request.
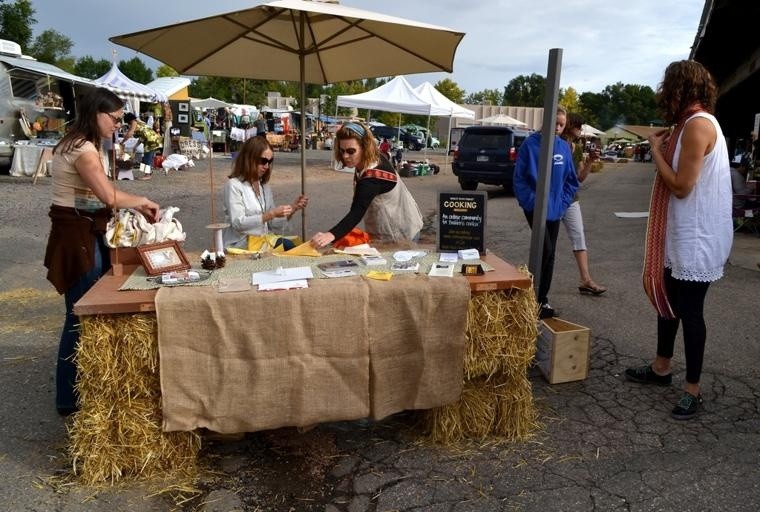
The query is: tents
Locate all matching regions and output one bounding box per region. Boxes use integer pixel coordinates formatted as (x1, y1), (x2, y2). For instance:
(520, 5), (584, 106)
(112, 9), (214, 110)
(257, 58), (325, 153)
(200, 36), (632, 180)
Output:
(92, 53), (167, 115)
(331, 74), (450, 170)
(414, 80), (474, 173)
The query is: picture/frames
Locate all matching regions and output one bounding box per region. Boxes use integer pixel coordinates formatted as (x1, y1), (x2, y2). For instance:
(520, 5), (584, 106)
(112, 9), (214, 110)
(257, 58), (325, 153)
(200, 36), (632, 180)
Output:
(179, 103), (188, 111)
(179, 114), (188, 123)
(137, 239), (192, 276)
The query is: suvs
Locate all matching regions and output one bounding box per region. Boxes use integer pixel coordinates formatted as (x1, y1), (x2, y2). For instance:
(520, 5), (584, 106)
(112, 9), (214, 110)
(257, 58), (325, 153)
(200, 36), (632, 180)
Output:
(451, 124), (533, 192)
(370, 125), (426, 151)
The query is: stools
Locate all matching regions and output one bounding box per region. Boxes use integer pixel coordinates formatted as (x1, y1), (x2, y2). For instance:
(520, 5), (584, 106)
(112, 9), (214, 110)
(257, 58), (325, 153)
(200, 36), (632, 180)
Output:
(117, 168), (135, 181)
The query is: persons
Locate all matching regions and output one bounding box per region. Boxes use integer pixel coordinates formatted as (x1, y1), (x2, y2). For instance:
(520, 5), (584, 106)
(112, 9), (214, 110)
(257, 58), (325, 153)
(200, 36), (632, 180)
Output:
(323, 136), (333, 150)
(203, 111), (211, 131)
(634, 145), (641, 161)
(45, 88), (161, 417)
(561, 112), (609, 294)
(640, 145), (647, 163)
(309, 121), (425, 248)
(514, 107), (580, 318)
(623, 60), (734, 420)
(379, 138), (391, 152)
(730, 157), (760, 210)
(391, 146), (402, 167)
(119, 113), (162, 180)
(254, 114), (266, 139)
(222, 136), (308, 251)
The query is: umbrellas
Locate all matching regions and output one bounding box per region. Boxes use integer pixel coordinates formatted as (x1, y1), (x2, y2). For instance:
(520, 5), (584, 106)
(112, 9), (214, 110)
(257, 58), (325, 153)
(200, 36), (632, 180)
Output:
(477, 112), (526, 128)
(109, 0), (468, 244)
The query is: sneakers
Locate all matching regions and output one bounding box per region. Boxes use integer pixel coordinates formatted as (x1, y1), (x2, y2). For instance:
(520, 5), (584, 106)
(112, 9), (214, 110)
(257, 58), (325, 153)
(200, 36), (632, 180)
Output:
(672, 392), (703, 419)
(625, 364), (673, 386)
(139, 174), (151, 179)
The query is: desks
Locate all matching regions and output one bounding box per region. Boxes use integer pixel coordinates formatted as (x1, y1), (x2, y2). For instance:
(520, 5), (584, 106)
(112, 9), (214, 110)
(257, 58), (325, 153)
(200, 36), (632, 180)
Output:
(9, 139), (110, 177)
(75, 243), (530, 479)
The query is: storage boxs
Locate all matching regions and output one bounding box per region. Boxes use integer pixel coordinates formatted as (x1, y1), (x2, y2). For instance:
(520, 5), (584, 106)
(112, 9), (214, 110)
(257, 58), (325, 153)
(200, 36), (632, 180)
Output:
(111, 241), (184, 264)
(534, 317), (589, 385)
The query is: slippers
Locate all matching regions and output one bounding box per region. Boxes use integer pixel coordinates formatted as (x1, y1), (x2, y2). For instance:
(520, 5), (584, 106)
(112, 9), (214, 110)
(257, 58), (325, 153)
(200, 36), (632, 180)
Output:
(579, 280), (607, 294)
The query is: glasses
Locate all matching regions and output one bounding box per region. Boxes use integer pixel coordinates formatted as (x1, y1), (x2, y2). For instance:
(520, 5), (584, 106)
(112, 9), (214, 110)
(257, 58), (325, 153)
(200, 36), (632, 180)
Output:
(259, 157), (274, 164)
(104, 112), (122, 124)
(339, 147), (356, 154)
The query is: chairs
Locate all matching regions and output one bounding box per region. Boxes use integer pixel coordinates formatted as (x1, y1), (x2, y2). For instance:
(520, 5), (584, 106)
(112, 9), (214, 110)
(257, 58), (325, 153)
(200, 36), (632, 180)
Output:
(733, 194), (760, 233)
